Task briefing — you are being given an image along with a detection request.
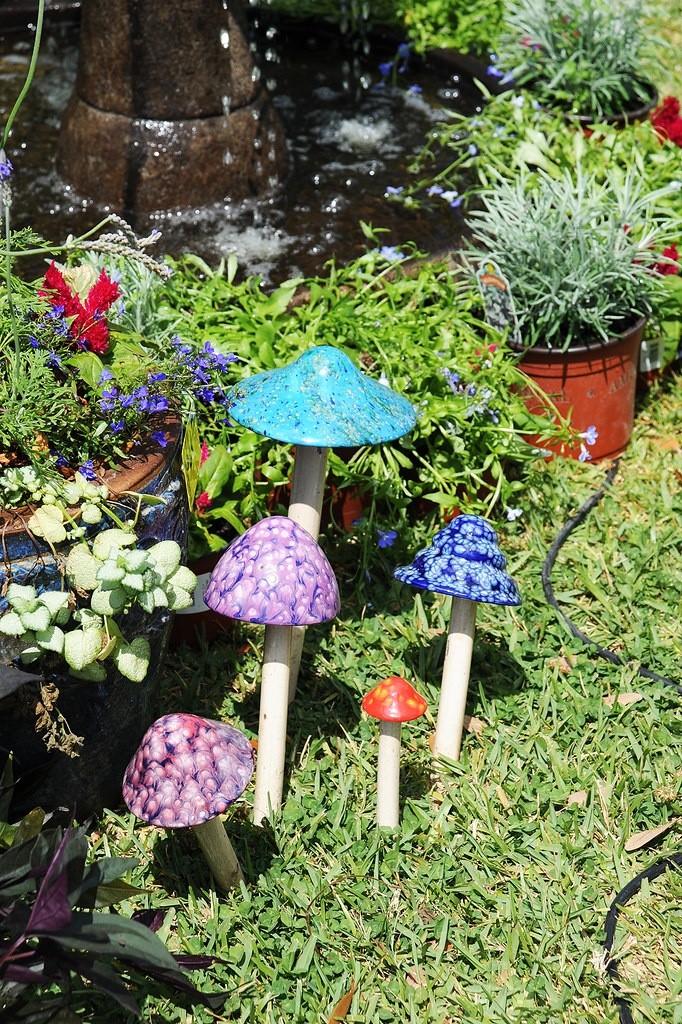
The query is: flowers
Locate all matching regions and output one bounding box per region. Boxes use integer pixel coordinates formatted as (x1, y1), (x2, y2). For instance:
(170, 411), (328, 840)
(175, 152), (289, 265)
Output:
(0, 0), (296, 687)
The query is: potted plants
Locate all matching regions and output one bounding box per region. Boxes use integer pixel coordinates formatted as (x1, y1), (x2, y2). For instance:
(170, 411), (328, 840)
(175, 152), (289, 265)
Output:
(496, 0), (672, 129)
(451, 160), (682, 465)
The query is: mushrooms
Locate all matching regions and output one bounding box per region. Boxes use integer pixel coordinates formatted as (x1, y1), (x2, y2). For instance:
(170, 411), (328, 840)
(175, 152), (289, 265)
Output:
(121, 345), (521, 891)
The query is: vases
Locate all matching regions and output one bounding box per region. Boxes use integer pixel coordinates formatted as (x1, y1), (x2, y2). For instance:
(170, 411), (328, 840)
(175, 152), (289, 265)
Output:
(0, 402), (190, 754)
